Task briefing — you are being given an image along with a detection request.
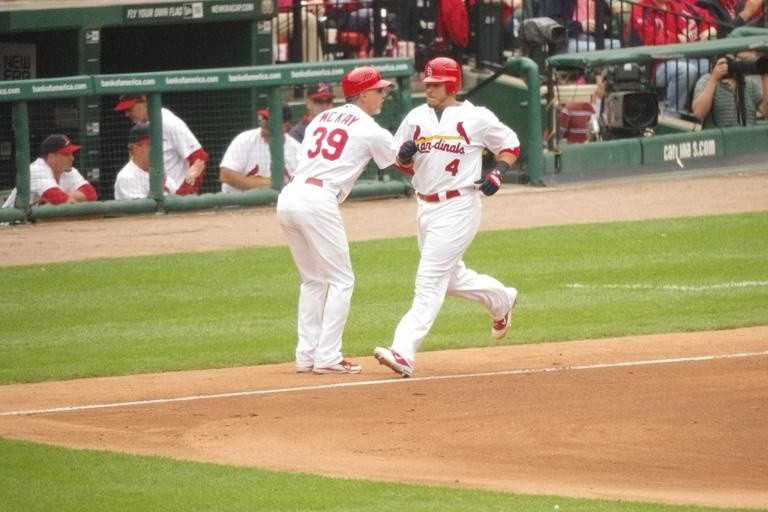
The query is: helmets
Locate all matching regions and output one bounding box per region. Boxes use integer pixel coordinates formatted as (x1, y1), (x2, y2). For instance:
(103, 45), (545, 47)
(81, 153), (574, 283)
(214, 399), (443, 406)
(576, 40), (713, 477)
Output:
(342, 67), (391, 97)
(422, 57), (461, 96)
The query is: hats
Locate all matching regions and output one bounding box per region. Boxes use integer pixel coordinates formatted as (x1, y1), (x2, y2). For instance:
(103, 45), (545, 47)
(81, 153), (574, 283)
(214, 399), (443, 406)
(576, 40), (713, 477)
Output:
(40, 135), (81, 155)
(128, 123), (152, 145)
(307, 82), (334, 98)
(257, 103), (291, 122)
(112, 94), (139, 111)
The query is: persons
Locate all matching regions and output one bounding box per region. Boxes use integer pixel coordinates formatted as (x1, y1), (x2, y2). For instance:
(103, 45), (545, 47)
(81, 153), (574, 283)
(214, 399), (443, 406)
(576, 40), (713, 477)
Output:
(1, 135), (98, 208)
(372, 56), (520, 378)
(220, 81), (337, 192)
(274, 0), (768, 145)
(113, 93), (209, 200)
(276, 67), (413, 376)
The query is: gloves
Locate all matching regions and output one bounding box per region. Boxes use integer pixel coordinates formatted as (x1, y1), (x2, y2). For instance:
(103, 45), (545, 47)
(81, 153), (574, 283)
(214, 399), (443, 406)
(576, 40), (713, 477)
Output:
(399, 140), (416, 165)
(475, 160), (509, 196)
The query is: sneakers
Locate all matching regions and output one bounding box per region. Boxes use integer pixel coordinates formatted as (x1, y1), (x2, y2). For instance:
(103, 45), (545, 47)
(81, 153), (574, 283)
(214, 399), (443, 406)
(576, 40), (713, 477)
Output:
(296, 366), (313, 372)
(313, 360), (362, 374)
(492, 287), (518, 339)
(374, 347), (412, 377)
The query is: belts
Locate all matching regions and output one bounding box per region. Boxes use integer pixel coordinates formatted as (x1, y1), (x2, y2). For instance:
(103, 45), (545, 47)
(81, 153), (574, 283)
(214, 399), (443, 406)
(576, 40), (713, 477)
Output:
(418, 191), (460, 202)
(305, 178), (323, 187)
